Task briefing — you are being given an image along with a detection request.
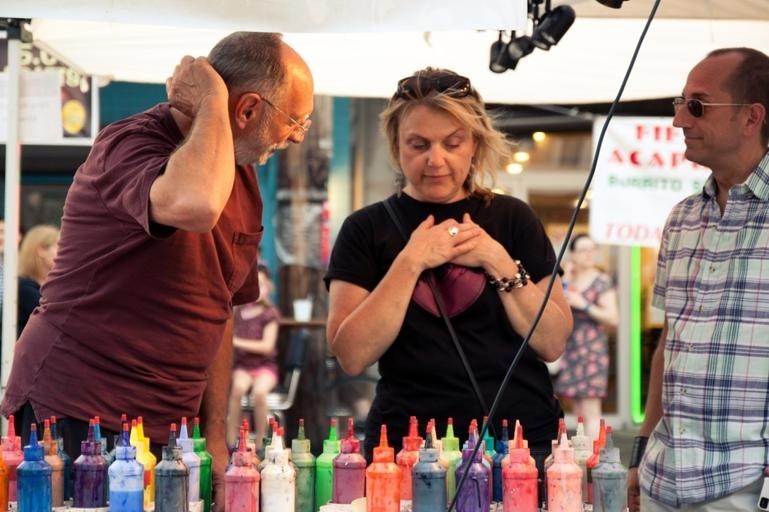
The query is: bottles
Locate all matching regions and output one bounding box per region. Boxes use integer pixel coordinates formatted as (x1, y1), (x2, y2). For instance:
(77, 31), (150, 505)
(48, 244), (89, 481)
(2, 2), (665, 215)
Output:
(0, 410), (631, 510)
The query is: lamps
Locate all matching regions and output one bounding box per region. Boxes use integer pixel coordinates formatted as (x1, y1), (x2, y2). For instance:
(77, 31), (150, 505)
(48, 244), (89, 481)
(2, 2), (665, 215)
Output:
(485, 1), (577, 74)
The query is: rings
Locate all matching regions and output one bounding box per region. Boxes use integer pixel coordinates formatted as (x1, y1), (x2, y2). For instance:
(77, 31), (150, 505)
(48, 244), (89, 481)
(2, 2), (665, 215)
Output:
(448, 226), (459, 236)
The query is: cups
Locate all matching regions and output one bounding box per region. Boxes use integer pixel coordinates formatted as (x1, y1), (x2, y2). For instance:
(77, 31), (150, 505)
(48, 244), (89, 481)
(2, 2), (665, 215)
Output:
(291, 298), (313, 323)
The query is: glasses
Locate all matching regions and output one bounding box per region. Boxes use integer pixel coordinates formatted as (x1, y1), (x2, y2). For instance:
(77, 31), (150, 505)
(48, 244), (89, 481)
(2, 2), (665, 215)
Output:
(395, 73), (474, 100)
(672, 95), (769, 126)
(257, 93), (316, 136)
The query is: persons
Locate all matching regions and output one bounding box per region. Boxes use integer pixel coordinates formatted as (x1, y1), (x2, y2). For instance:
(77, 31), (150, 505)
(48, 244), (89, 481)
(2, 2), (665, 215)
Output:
(550, 231), (622, 437)
(321, 66), (573, 509)
(626, 47), (769, 511)
(18, 223), (59, 337)
(0, 216), (25, 326)
(0, 30), (315, 512)
(226, 264), (281, 459)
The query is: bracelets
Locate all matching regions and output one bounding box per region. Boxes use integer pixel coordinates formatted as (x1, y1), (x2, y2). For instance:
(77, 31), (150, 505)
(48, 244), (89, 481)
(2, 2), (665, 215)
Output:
(583, 300), (590, 314)
(629, 436), (649, 468)
(486, 260), (530, 293)
